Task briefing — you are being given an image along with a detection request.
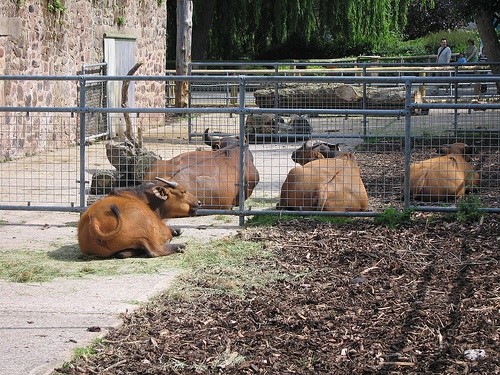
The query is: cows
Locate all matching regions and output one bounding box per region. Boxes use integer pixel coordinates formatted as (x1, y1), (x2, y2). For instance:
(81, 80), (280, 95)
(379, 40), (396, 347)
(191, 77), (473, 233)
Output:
(76, 176), (202, 260)
(141, 126), (261, 210)
(402, 140), (481, 203)
(274, 137), (370, 213)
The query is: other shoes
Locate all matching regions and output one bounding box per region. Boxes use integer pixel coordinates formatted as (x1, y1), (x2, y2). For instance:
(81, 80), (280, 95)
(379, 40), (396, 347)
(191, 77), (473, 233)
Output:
(431, 91), (438, 94)
(444, 91), (448, 94)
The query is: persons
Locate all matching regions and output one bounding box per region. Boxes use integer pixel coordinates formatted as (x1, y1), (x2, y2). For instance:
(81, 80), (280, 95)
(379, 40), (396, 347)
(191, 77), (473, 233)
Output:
(456, 52), (467, 63)
(465, 38), (478, 86)
(434, 37), (451, 95)
(478, 47), (490, 94)
